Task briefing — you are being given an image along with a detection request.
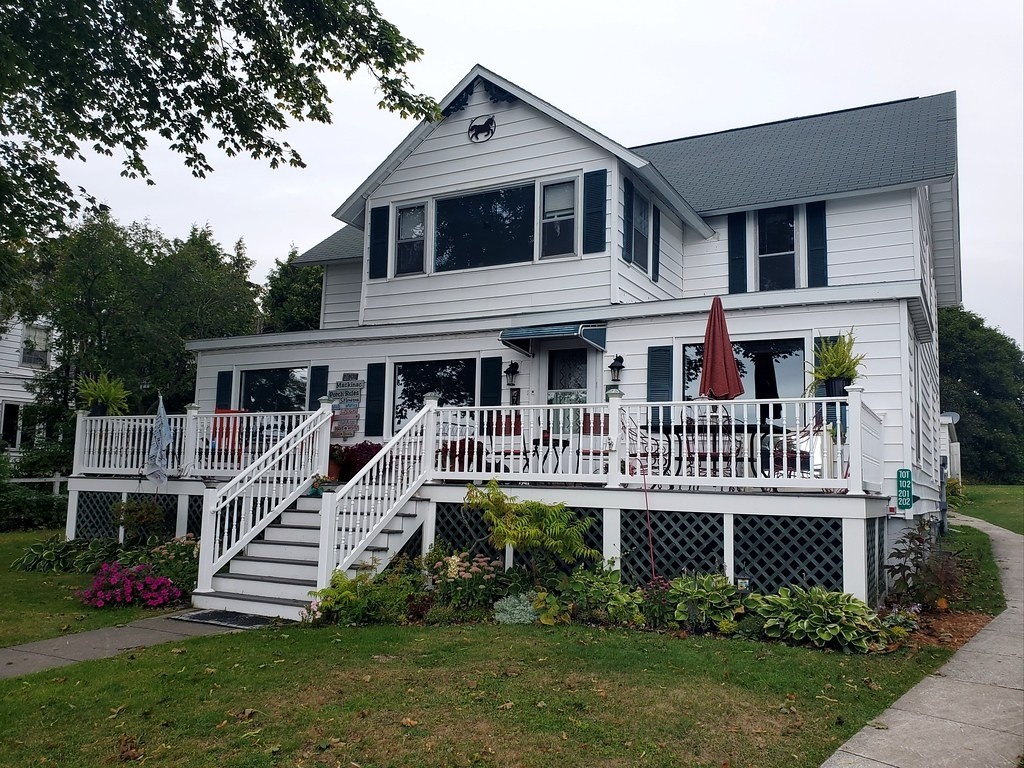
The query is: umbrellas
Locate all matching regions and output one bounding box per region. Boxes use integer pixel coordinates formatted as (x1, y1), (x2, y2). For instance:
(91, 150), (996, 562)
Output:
(698, 297), (745, 476)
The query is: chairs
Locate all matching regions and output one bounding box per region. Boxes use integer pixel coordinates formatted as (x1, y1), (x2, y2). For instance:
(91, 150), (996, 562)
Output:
(759, 405), (822, 492)
(488, 422), (530, 472)
(576, 450), (609, 474)
(681, 397), (745, 492)
(620, 409), (671, 489)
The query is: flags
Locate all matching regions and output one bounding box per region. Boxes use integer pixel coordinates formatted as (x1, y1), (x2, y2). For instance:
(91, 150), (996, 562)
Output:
(146, 396), (173, 490)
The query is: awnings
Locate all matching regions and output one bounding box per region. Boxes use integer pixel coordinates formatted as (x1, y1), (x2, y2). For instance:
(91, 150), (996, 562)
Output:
(498, 322), (610, 358)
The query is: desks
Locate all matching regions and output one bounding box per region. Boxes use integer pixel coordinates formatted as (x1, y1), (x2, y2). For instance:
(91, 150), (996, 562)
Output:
(640, 424), (779, 492)
(533, 439), (570, 474)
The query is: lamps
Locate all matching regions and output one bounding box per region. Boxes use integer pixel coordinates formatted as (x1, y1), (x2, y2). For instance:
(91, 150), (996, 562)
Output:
(608, 354), (625, 381)
(504, 360), (520, 386)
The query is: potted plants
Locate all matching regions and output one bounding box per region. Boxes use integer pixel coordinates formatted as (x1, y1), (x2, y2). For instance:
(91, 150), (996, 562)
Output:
(435, 438), (490, 484)
(801, 325), (868, 406)
(827, 421), (847, 461)
(343, 440), (393, 485)
(328, 443), (344, 481)
